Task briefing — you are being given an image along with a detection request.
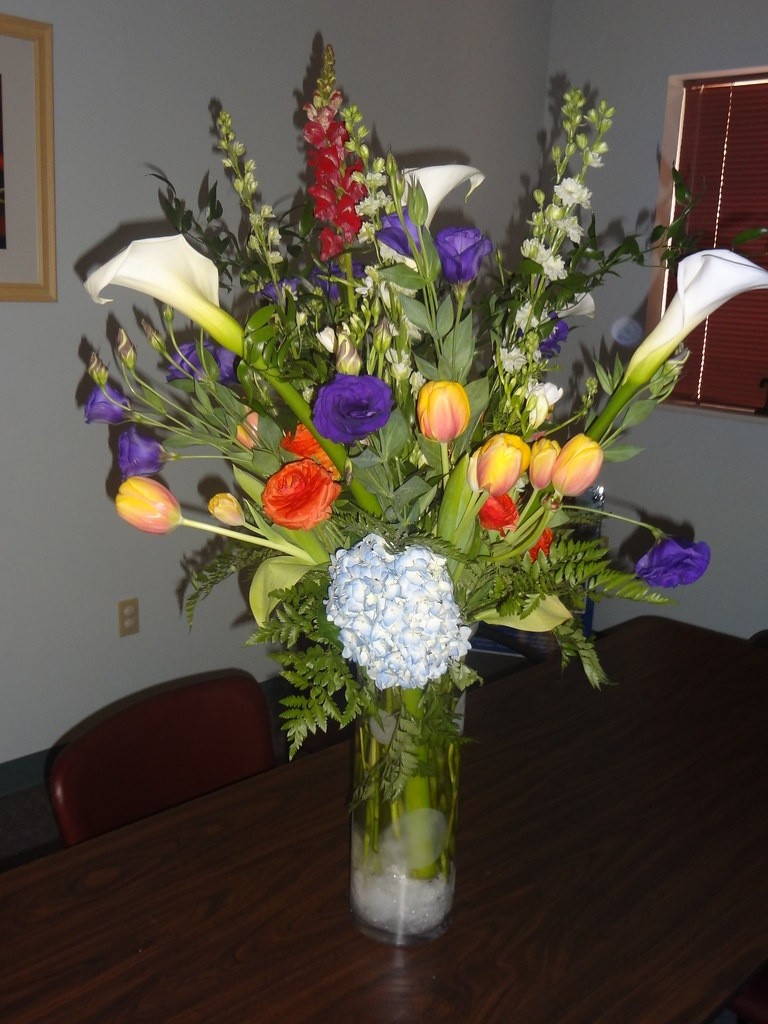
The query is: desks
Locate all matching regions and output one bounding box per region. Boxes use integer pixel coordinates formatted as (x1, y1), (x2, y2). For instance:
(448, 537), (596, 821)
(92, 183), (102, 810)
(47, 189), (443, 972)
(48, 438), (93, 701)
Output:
(0, 614), (766, 1023)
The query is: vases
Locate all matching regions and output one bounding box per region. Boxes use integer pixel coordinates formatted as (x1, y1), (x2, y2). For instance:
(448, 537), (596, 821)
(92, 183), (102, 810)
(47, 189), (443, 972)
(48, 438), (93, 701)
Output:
(345, 661), (470, 938)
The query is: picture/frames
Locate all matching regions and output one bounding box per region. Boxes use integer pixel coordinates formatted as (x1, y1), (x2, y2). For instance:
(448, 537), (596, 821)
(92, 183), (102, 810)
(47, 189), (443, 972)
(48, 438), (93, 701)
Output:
(0, 15), (57, 304)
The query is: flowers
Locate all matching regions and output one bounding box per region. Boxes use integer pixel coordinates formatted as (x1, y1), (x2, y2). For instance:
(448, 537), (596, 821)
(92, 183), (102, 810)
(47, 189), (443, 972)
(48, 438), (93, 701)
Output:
(75, 44), (764, 872)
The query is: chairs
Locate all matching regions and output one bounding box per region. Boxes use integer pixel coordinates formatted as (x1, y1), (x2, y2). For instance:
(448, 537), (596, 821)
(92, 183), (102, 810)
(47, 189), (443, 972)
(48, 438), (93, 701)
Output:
(45, 670), (278, 849)
